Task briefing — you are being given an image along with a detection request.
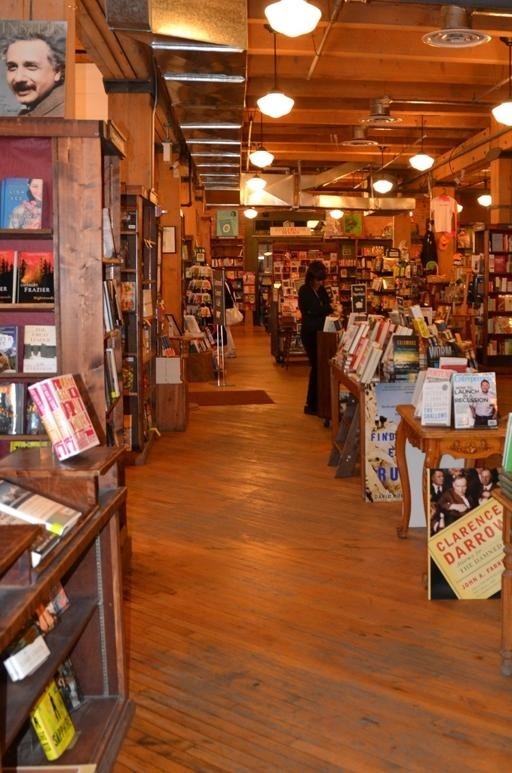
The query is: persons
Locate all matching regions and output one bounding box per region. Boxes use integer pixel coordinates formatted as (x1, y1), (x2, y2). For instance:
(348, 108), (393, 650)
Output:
(428, 467), (497, 537)
(297, 260), (336, 416)
(225, 282), (234, 354)
(1, 21), (68, 117)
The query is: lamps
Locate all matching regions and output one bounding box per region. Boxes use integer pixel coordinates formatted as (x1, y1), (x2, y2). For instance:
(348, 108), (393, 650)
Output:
(329, 209), (345, 220)
(243, 207), (259, 220)
(255, 24), (295, 119)
(372, 146), (394, 195)
(249, 112), (275, 169)
(456, 203), (463, 214)
(408, 115), (435, 172)
(246, 173), (267, 192)
(476, 176), (493, 207)
(263, 1), (323, 39)
(490, 36), (512, 128)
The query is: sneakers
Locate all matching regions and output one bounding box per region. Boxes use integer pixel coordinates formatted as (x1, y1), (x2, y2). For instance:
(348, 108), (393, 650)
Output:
(304, 405), (317, 414)
(224, 352), (237, 358)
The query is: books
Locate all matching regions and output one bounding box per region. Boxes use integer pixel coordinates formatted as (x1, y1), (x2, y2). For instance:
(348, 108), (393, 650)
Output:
(100, 209), (223, 451)
(211, 248), (255, 309)
(1, 179), (101, 763)
(327, 229), (511, 473)
(258, 249), (338, 353)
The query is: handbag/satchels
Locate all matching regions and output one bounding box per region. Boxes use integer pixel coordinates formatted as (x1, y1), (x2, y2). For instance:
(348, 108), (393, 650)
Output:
(225, 308), (244, 326)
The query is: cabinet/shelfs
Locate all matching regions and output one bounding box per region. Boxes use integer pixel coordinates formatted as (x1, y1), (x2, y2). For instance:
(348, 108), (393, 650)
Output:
(252, 214), (512, 374)
(0, 117), (105, 450)
(0, 442), (139, 773)
(316, 329), (512, 679)
(120, 185), (246, 465)
